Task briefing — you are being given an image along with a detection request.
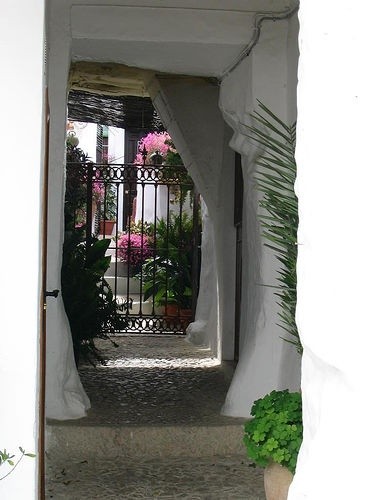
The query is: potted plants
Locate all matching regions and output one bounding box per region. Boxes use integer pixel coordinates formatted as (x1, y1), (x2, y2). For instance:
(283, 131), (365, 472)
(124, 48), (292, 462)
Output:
(160, 219), (200, 334)
(242, 390), (305, 500)
(140, 213), (186, 321)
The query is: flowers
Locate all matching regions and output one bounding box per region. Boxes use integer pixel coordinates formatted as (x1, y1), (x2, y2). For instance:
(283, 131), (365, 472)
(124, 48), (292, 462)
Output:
(115, 232), (149, 264)
(95, 168), (119, 220)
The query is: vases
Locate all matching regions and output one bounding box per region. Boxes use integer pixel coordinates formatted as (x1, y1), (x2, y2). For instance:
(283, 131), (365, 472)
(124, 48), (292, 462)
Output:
(97, 220), (116, 235)
